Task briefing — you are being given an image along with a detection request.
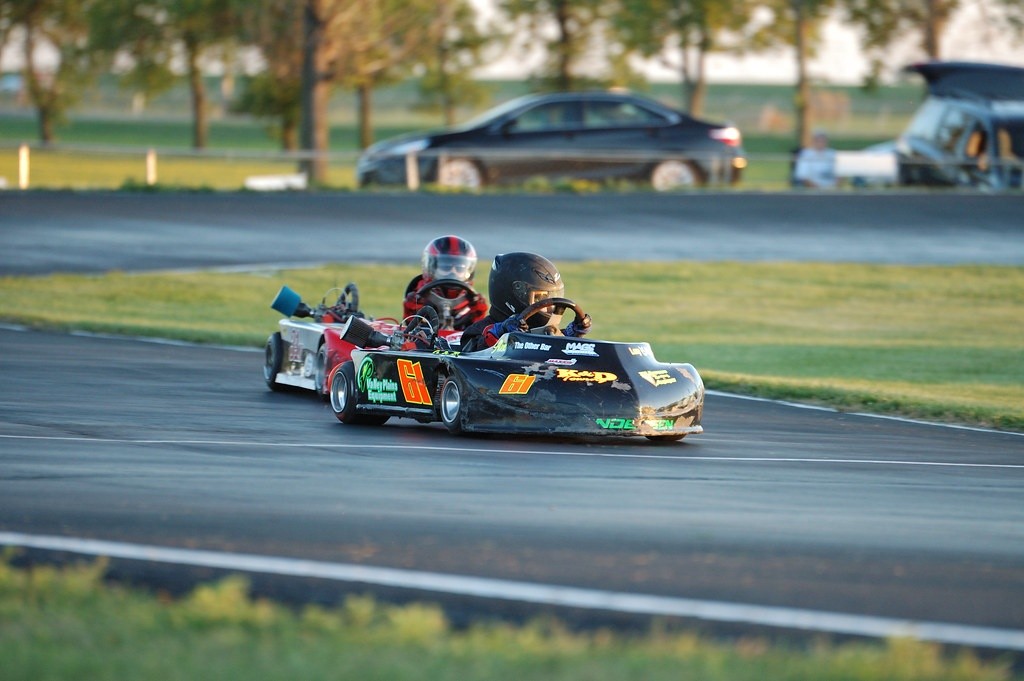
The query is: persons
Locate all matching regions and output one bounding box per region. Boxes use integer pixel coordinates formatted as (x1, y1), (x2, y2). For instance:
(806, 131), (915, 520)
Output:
(460, 252), (592, 353)
(403, 236), (488, 331)
(795, 130), (837, 188)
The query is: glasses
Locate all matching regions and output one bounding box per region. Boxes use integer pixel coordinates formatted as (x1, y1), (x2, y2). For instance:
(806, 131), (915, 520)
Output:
(534, 293), (548, 303)
(437, 260), (466, 273)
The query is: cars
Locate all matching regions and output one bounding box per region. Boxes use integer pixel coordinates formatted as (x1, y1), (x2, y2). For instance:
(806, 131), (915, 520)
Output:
(354, 89), (747, 193)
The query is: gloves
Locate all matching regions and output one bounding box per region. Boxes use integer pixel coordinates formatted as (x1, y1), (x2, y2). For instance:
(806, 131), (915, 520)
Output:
(562, 314), (592, 337)
(488, 314), (529, 340)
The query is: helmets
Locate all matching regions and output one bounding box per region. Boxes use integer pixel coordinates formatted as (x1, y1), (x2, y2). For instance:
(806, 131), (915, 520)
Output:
(489, 252), (566, 334)
(423, 235), (476, 290)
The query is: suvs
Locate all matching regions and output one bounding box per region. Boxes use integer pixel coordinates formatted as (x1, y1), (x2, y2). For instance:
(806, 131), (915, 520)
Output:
(895, 62), (1023, 191)
